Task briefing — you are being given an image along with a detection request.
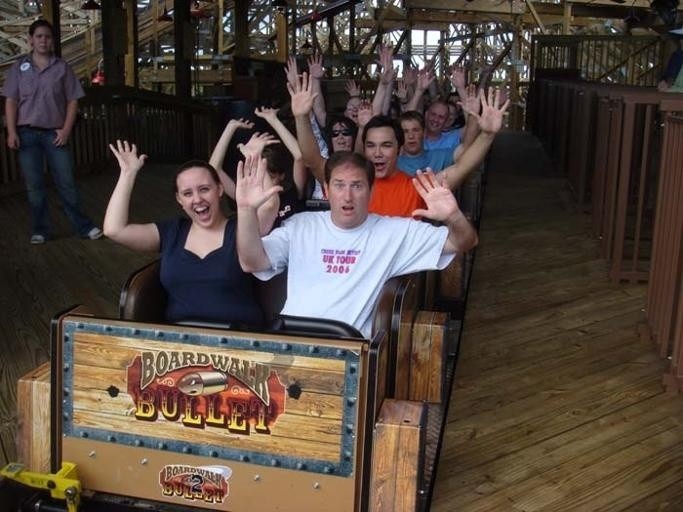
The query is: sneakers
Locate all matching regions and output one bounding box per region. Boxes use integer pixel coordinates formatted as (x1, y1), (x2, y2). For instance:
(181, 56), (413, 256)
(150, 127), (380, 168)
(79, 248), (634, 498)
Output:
(30, 233), (45, 245)
(84, 226), (104, 241)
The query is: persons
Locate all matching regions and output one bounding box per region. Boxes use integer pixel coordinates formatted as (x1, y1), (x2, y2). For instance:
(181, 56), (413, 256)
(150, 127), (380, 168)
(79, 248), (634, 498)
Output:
(235, 150), (480, 341)
(208, 43), (512, 229)
(0, 18), (102, 246)
(656, 48), (683, 94)
(101, 131), (282, 333)
